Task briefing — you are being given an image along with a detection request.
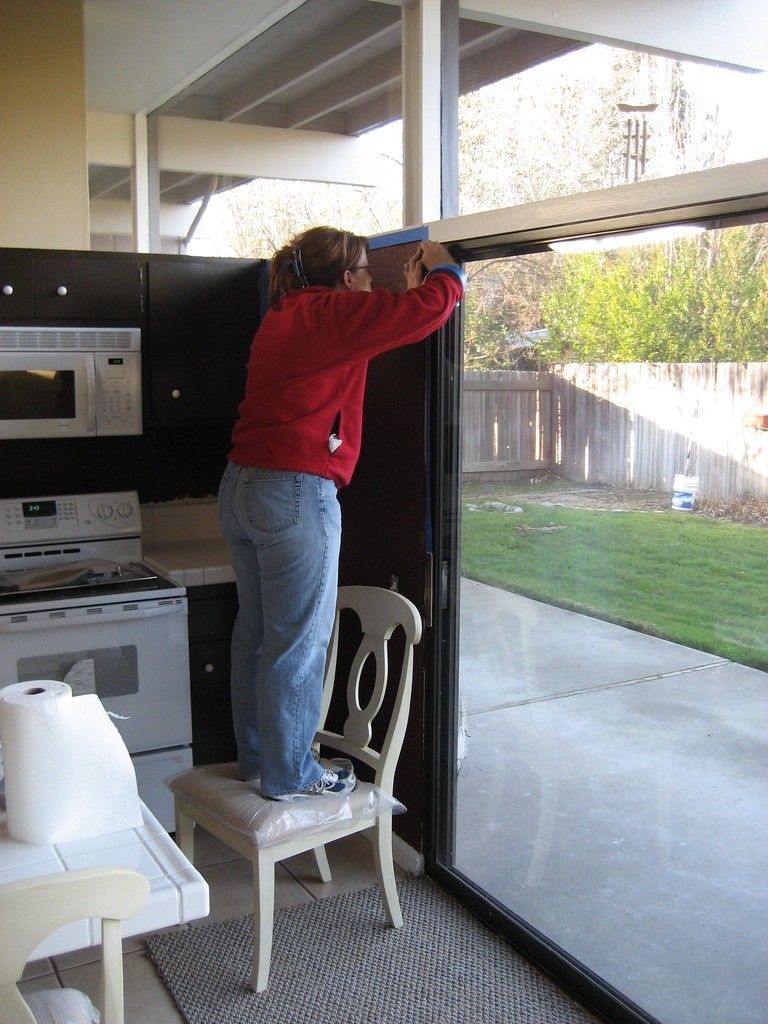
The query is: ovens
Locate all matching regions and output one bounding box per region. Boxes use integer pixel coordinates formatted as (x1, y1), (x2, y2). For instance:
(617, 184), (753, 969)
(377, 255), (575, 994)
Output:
(0, 599), (196, 833)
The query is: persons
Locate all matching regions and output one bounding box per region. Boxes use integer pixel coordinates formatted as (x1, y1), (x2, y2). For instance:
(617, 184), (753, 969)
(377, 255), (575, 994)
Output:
(218, 227), (467, 799)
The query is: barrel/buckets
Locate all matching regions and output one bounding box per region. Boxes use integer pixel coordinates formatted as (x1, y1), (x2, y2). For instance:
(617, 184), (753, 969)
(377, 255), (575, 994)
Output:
(671, 473), (700, 511)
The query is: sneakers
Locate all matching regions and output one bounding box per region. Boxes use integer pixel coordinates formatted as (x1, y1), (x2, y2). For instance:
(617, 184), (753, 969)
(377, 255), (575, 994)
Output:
(261, 769), (357, 802)
(311, 748), (320, 764)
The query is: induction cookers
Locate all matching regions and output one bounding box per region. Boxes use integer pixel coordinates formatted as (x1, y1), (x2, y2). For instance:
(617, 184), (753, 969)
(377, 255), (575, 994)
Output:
(0, 490), (187, 614)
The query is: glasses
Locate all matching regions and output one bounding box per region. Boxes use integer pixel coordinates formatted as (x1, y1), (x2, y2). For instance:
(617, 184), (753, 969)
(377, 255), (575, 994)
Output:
(347, 264), (375, 276)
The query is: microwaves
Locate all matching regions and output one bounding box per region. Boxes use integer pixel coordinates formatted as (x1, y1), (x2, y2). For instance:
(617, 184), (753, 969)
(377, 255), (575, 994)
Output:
(0, 327), (143, 440)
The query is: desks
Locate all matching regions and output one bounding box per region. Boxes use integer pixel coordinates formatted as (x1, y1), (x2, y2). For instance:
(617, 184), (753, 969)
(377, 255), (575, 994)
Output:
(0, 795), (209, 1024)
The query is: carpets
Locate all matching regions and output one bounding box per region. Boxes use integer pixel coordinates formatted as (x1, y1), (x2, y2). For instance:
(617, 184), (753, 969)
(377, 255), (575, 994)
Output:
(146, 873), (607, 1024)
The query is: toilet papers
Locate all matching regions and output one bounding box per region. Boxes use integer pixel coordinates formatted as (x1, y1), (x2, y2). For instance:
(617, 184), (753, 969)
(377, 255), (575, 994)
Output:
(2, 678), (145, 846)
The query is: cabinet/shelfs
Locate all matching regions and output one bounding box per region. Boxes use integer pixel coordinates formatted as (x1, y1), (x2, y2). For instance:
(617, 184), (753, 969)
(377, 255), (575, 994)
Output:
(186, 582), (240, 770)
(0, 248), (260, 428)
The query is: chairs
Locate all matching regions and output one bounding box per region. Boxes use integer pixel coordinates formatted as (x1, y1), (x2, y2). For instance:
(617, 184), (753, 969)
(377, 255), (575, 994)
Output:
(0, 865), (150, 1024)
(171, 586), (422, 995)
